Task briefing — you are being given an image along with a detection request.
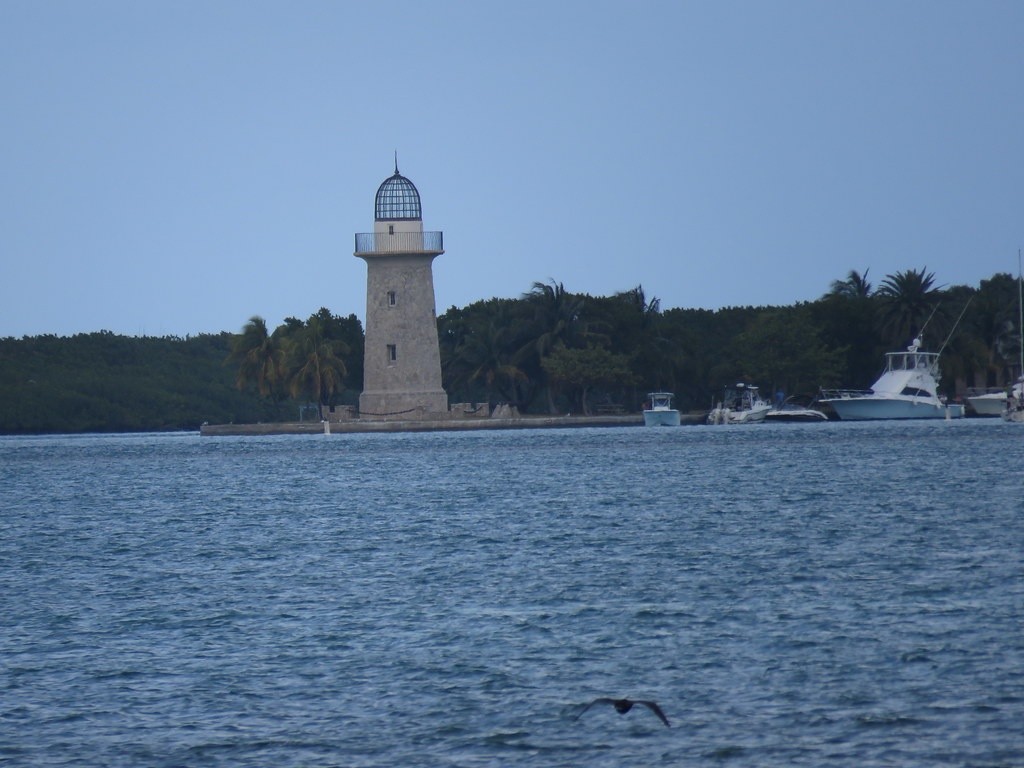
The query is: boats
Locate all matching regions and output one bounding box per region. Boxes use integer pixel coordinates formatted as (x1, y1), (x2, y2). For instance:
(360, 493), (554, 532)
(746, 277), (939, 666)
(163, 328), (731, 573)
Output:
(969, 376), (1024, 418)
(766, 409), (830, 423)
(707, 383), (771, 426)
(641, 390), (682, 427)
(818, 351), (966, 419)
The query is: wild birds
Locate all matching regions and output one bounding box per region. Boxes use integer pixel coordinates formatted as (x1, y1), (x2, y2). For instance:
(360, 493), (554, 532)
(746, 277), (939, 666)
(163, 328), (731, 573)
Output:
(574, 697), (671, 729)
